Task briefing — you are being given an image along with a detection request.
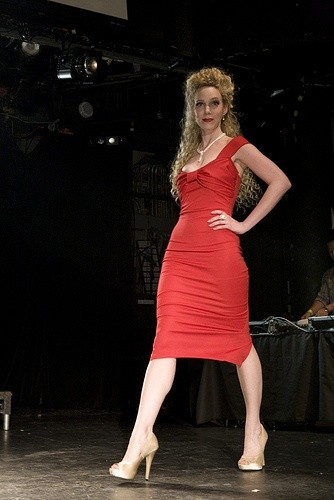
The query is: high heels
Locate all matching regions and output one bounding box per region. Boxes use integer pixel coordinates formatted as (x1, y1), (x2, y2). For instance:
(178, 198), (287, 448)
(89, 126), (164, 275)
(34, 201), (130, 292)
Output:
(238, 424), (268, 470)
(109, 432), (160, 479)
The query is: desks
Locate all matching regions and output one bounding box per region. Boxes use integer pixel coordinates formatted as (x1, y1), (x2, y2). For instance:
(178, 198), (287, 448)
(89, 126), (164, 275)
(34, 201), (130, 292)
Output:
(195, 329), (334, 431)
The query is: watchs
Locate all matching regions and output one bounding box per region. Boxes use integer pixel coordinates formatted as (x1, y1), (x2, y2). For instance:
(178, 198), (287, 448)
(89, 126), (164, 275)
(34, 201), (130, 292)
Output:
(324, 308), (328, 312)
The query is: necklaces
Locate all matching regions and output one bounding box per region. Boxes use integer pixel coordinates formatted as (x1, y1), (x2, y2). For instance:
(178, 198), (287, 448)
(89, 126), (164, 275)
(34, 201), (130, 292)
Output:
(196, 133), (225, 163)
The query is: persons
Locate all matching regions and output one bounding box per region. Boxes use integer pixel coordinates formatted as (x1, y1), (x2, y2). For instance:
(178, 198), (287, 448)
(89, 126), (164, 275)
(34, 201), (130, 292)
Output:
(109, 66), (292, 481)
(300, 236), (334, 319)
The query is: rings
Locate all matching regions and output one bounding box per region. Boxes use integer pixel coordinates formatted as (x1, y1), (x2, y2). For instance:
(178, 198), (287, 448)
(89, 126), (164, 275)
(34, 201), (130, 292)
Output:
(220, 214), (227, 219)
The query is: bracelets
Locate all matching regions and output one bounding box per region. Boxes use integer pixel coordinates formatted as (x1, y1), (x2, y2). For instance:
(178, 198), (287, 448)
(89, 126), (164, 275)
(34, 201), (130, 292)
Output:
(307, 309), (314, 316)
